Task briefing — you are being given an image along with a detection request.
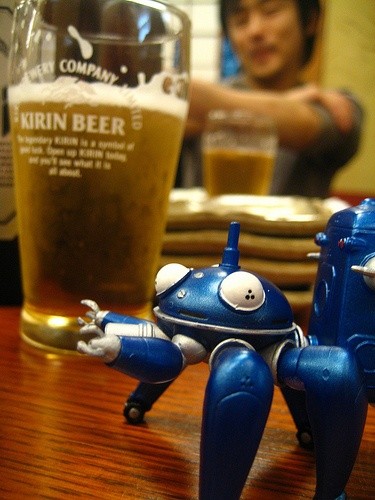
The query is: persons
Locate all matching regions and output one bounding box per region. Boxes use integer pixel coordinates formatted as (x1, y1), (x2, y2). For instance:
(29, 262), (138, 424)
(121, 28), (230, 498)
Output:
(179, 0), (363, 197)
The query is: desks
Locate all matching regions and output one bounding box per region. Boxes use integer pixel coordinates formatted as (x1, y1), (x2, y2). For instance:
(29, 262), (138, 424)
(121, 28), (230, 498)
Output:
(0, 304), (375, 500)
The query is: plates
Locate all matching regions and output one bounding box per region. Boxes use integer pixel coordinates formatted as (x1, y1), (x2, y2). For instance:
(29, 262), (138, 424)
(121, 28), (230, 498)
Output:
(157, 187), (350, 311)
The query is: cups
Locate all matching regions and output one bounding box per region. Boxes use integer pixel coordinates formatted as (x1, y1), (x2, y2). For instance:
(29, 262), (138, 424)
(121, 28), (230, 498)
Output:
(8, 0), (193, 356)
(202, 109), (278, 199)
